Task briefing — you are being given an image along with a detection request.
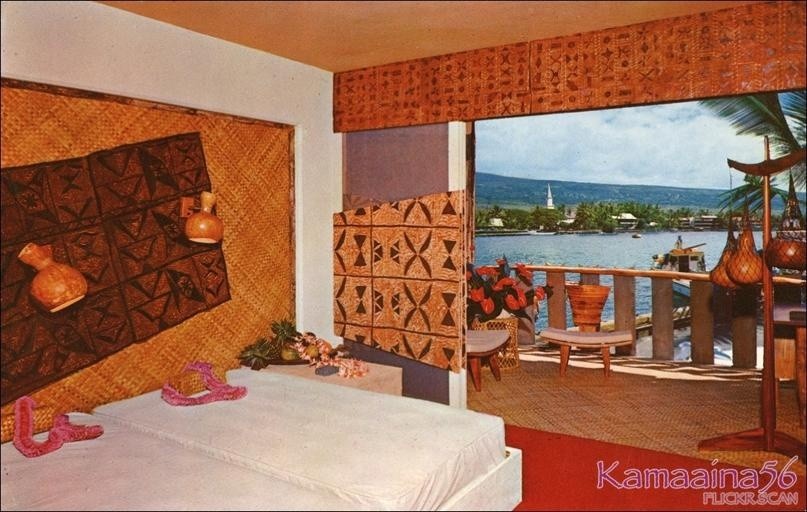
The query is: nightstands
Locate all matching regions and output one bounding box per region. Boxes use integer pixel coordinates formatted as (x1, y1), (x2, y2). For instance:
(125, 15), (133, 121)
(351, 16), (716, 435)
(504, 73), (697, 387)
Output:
(259, 358), (402, 397)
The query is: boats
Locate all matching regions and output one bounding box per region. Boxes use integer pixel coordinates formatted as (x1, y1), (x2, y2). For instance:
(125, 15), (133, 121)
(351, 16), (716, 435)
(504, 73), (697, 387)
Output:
(632, 233), (642, 238)
(651, 234), (707, 298)
(650, 250), (707, 297)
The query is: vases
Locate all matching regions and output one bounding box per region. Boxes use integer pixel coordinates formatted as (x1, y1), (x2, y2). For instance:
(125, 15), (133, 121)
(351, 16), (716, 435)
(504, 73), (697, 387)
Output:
(472, 317), (519, 369)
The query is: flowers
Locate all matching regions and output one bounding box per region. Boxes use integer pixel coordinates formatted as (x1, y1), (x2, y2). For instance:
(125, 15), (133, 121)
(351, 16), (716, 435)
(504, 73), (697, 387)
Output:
(466, 256), (552, 321)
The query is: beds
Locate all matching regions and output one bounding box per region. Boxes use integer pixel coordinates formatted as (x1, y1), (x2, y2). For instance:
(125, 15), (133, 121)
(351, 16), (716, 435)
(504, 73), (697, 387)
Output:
(0, 368), (524, 510)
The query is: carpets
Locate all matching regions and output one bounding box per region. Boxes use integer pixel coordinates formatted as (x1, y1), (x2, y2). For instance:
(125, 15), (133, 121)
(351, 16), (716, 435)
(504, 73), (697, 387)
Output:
(501, 424), (807, 510)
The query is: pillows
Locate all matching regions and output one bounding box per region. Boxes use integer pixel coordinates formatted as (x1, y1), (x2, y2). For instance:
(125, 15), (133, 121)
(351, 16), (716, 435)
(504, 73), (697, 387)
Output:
(0, 408), (58, 445)
(167, 364), (226, 398)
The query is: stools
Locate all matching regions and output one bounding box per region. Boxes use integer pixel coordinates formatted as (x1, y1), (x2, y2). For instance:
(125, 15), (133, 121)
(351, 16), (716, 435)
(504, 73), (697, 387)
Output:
(541, 326), (632, 377)
(468, 330), (510, 391)
